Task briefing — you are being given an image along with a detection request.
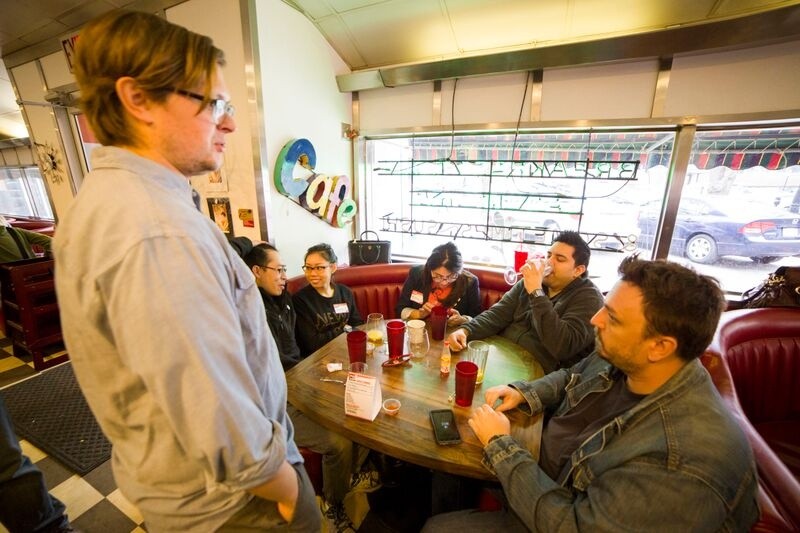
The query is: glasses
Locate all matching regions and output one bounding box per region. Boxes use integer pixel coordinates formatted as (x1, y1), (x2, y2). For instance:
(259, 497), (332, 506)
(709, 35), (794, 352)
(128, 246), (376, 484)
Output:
(430, 270), (458, 282)
(257, 264), (287, 273)
(164, 86), (236, 124)
(301, 265), (331, 271)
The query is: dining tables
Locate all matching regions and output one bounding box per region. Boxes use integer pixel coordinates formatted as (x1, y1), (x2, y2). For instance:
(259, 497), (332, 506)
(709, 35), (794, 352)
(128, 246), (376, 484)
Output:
(286, 318), (546, 515)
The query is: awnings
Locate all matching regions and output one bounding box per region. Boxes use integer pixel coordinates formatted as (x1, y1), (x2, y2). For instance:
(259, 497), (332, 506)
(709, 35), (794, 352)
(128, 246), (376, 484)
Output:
(410, 127), (800, 170)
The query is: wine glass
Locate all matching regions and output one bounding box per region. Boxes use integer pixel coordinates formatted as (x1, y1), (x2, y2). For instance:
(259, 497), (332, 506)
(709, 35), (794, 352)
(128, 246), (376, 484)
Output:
(504, 259), (552, 285)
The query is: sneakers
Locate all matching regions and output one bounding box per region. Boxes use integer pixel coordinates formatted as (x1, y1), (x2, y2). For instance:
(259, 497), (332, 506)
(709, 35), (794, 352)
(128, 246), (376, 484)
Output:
(348, 470), (383, 495)
(319, 496), (359, 532)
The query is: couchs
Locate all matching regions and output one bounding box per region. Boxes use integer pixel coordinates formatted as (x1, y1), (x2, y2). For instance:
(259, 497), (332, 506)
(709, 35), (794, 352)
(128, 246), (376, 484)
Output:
(697, 306), (800, 533)
(285, 262), (797, 533)
(4, 217), (56, 257)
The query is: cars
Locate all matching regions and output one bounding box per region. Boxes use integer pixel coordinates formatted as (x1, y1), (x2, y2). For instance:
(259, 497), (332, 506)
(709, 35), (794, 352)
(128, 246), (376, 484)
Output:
(639, 191), (800, 264)
(492, 182), (640, 249)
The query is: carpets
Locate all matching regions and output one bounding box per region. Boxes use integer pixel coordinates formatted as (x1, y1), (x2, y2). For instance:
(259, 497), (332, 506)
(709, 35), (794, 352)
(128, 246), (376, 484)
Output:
(0, 362), (114, 477)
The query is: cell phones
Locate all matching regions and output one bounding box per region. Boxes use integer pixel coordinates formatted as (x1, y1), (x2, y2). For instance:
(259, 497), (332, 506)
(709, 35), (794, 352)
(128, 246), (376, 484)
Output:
(429, 409), (463, 446)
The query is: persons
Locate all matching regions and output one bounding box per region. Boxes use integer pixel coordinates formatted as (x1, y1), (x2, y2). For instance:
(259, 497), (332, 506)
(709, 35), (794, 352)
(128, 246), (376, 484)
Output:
(417, 253), (763, 533)
(52, 9), (323, 533)
(446, 231), (609, 366)
(290, 241), (404, 469)
(1, 392), (82, 533)
(394, 242), (482, 327)
(229, 236), (382, 533)
(0, 215), (53, 262)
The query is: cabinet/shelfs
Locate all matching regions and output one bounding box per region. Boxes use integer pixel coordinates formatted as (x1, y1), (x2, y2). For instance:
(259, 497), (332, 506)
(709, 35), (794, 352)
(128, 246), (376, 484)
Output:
(0, 257), (71, 371)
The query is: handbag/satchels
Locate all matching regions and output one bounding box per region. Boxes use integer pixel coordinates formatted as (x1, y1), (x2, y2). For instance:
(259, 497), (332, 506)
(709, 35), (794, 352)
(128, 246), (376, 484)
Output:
(347, 230), (390, 265)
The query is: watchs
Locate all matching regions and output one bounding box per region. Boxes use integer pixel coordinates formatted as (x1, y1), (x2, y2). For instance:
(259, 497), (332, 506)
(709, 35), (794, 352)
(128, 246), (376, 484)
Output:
(529, 288), (545, 300)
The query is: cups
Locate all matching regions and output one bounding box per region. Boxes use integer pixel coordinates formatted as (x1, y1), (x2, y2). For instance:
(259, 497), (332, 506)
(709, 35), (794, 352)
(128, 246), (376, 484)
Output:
(345, 304), (493, 411)
